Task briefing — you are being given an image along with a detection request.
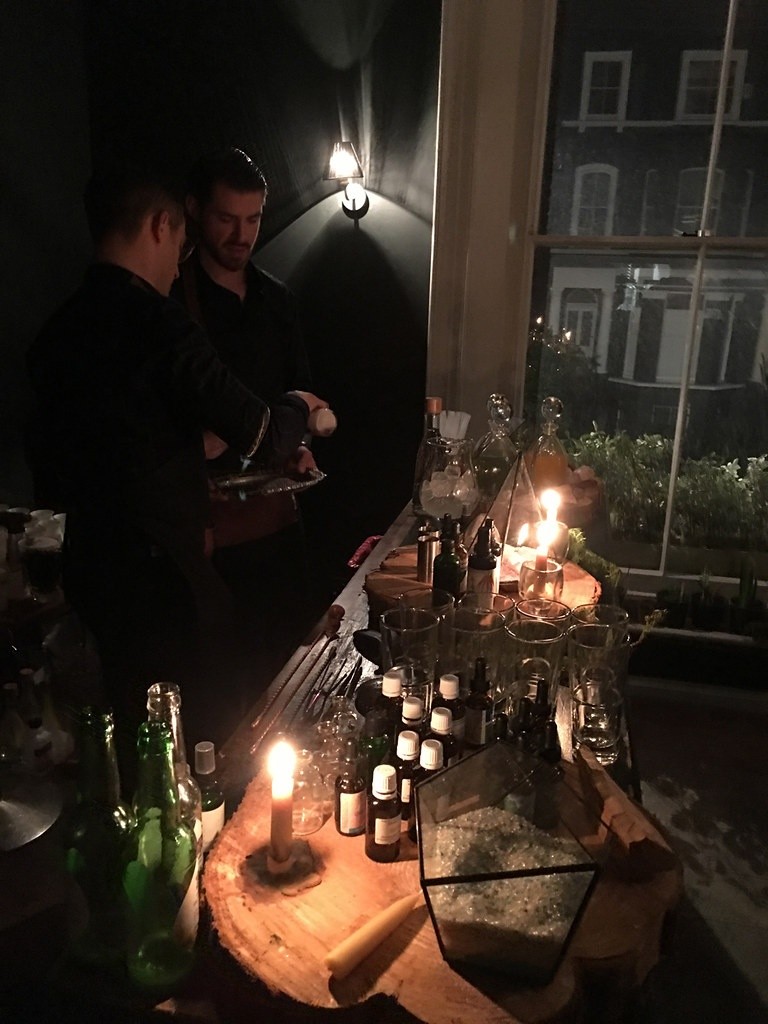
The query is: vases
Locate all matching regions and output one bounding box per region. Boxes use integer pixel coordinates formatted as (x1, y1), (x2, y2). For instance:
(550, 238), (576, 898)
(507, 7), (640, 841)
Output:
(607, 535), (768, 579)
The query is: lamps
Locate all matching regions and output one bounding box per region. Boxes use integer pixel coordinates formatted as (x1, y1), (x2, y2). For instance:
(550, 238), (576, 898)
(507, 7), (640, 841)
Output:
(324, 139), (370, 215)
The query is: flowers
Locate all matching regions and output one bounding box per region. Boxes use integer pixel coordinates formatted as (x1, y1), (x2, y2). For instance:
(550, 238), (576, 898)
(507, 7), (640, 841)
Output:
(562, 418), (768, 548)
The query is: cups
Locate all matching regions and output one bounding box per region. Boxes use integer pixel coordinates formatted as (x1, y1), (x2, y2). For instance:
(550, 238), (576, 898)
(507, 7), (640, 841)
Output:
(379, 587), (629, 766)
(419, 437), (480, 519)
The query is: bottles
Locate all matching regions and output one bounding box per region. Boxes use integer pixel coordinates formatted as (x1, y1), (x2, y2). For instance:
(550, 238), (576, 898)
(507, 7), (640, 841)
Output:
(287, 654), (557, 873)
(412, 396), (448, 519)
(433, 517), (504, 608)
(0, 666), (229, 1001)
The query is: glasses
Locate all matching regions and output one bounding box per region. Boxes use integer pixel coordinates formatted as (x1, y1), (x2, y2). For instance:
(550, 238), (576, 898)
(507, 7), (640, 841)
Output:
(173, 219), (197, 263)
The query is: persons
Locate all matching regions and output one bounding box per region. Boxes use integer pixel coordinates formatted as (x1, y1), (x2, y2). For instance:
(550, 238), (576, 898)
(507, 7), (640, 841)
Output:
(169, 151), (324, 653)
(18, 182), (329, 804)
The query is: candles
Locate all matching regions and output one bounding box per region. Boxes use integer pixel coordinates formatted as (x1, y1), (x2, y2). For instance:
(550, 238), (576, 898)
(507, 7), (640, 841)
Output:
(533, 544), (550, 594)
(545, 509), (557, 530)
(268, 740), (297, 863)
(323, 889), (424, 983)
